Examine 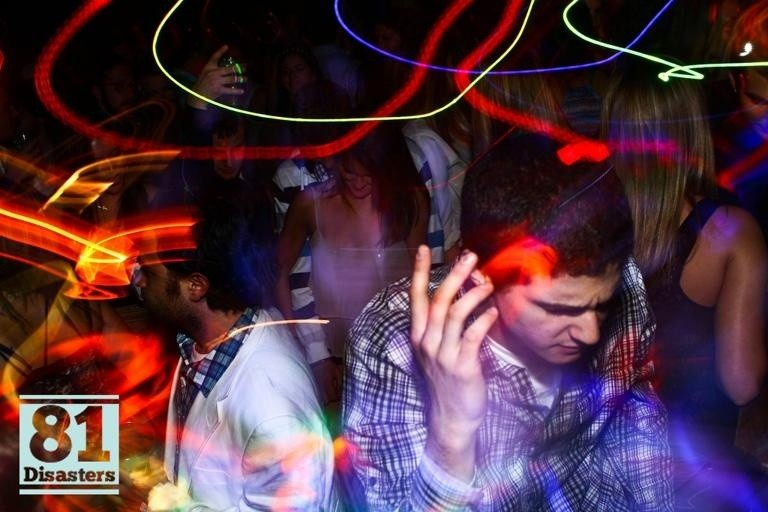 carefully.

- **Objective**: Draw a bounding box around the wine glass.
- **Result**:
[222,56,248,108]
